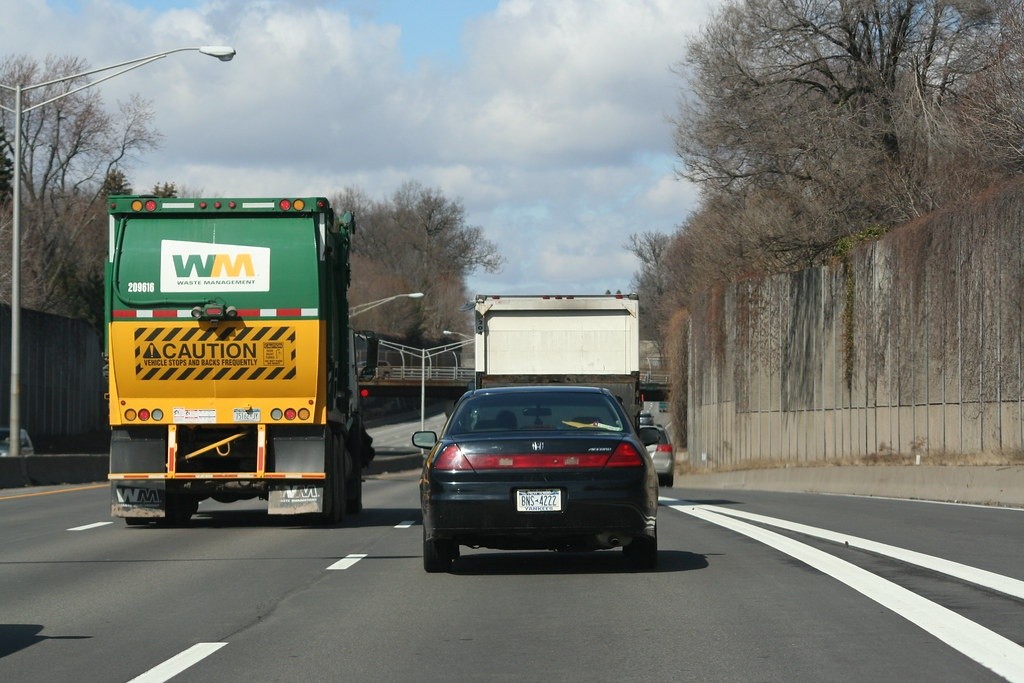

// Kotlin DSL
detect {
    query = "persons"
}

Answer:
[495,410,518,430]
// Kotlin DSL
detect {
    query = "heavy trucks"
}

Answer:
[101,197,381,526]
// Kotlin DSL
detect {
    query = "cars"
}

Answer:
[638,423,674,488]
[410,384,662,573]
[640,412,654,427]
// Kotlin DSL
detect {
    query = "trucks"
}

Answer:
[458,294,643,442]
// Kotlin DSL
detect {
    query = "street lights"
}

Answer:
[1,43,236,457]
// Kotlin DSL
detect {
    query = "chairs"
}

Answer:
[568,415,604,428]
[473,417,511,430]
